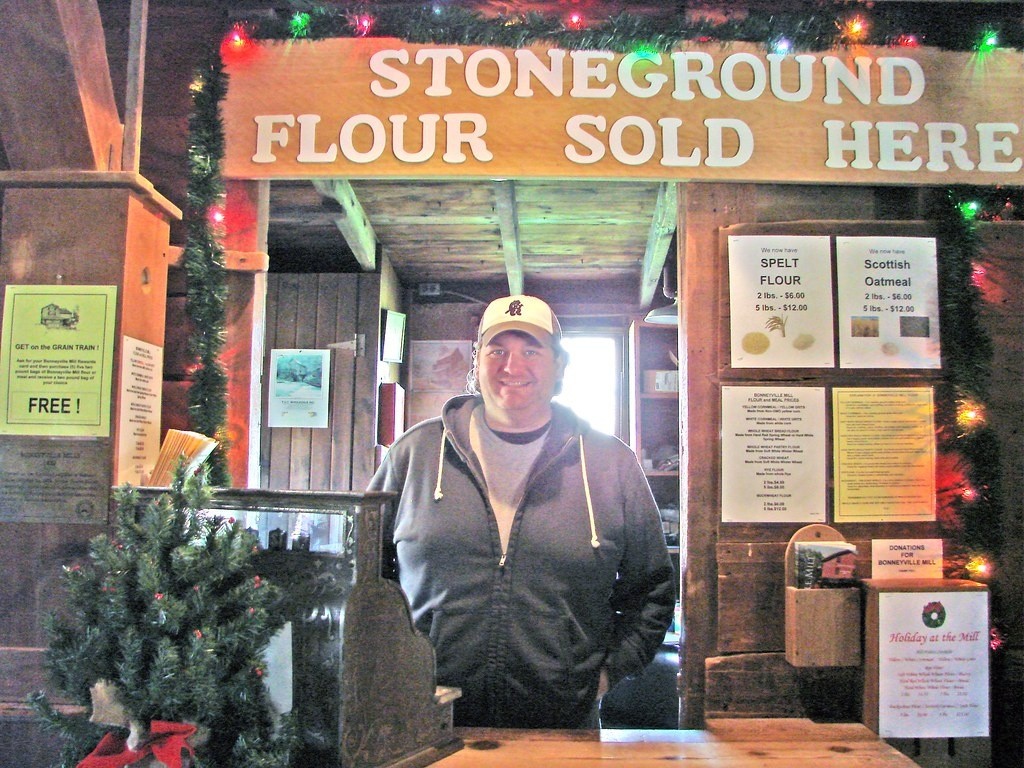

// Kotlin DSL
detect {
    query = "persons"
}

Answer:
[367,294,677,731]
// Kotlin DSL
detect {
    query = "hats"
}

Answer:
[477,294,562,353]
[652,445,679,471]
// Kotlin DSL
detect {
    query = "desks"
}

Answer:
[424,723,922,768]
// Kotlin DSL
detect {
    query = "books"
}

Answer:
[794,542,858,588]
